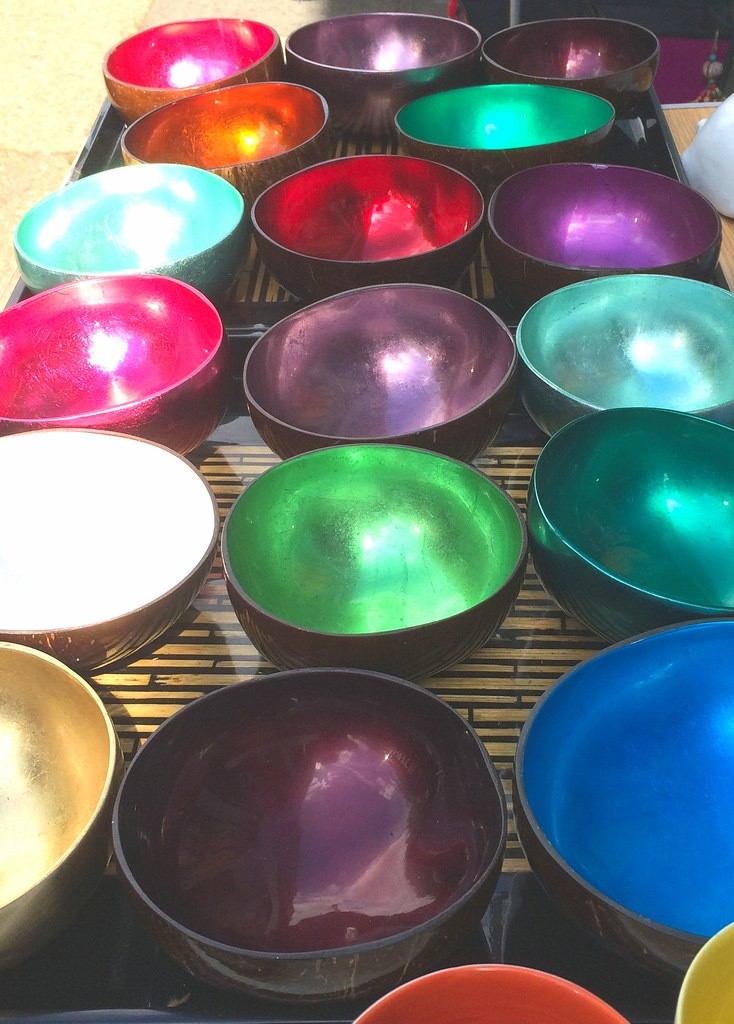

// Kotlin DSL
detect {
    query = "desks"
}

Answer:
[658,93,734,304]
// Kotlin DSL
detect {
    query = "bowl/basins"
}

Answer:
[393,82,617,207]
[249,153,487,305]
[285,11,483,140]
[102,17,285,125]
[514,272,734,437]
[525,406,734,647]
[241,282,520,466]
[487,161,723,318]
[1,273,232,458]
[0,427,222,675]
[11,162,252,316]
[0,615,734,1024]
[120,80,335,232]
[481,16,660,119]
[219,441,530,685]
